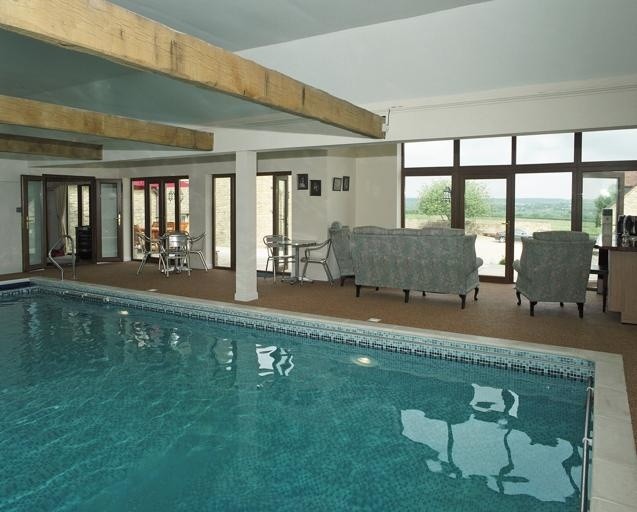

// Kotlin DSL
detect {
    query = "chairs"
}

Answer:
[328,220,380,292]
[261,234,301,284]
[298,238,335,288]
[135,230,210,277]
[511,230,596,321]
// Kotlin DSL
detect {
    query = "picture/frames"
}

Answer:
[296,173,351,196]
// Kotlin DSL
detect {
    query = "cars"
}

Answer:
[495,227,534,243]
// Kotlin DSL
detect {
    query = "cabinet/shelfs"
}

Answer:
[593,246,637,328]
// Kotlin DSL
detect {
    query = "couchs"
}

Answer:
[348,222,484,309]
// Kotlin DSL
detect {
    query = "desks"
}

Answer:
[277,239,317,285]
[574,263,608,313]
